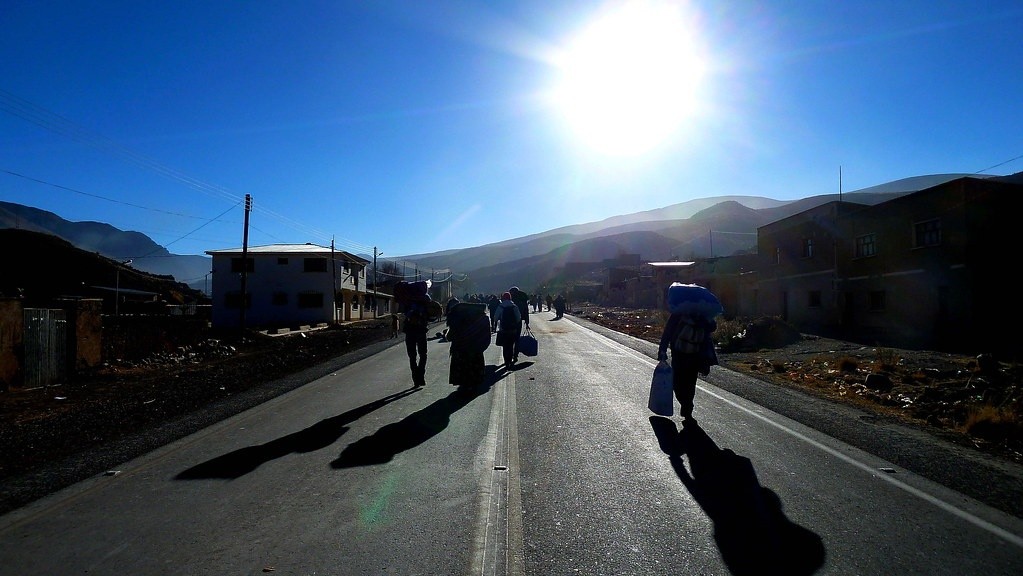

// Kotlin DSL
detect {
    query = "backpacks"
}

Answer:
[499,304,517,331]
[673,315,705,354]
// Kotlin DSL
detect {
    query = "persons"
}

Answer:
[530,293,565,319]
[657,313,716,418]
[446,314,491,385]
[509,286,530,354]
[493,293,521,369]
[435,292,501,333]
[395,280,442,388]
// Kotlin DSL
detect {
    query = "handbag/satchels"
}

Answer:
[518,329,538,356]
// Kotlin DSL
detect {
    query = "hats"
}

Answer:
[502,292,511,300]
